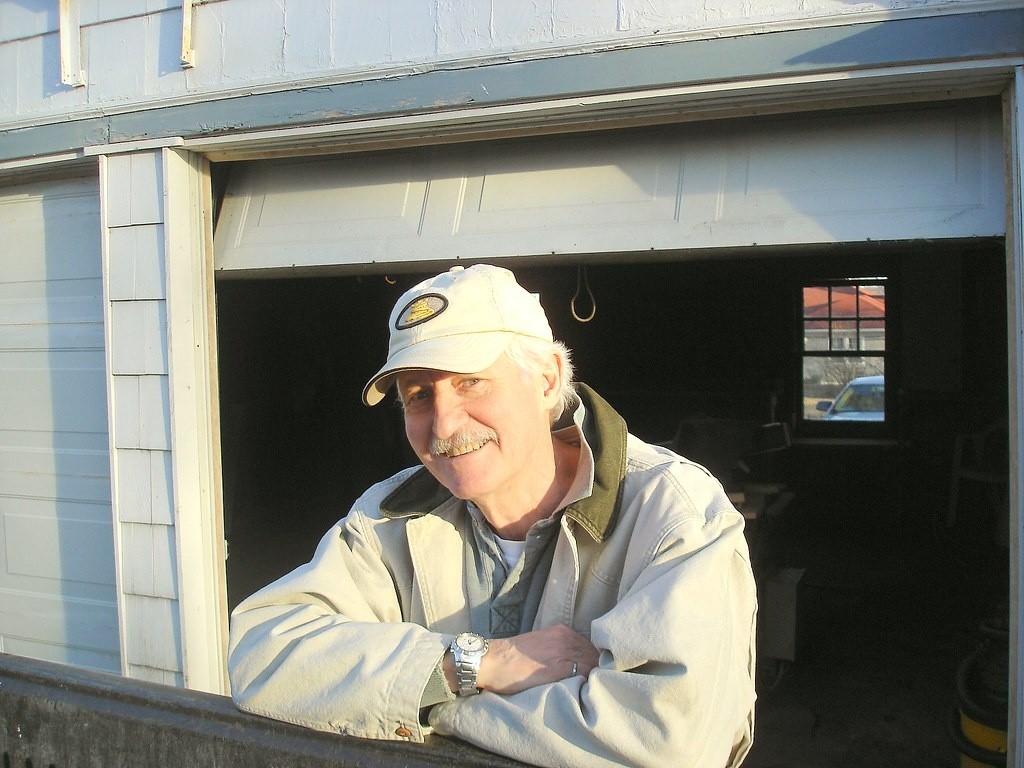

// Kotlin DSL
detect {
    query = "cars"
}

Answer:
[815,374,885,420]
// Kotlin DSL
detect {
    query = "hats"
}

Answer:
[362,263,553,407]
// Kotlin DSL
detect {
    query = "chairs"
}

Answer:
[946,414,1009,549]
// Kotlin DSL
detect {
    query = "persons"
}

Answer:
[229,265,759,768]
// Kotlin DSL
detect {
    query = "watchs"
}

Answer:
[450,632,490,697]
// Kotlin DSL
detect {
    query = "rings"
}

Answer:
[573,662,577,676]
